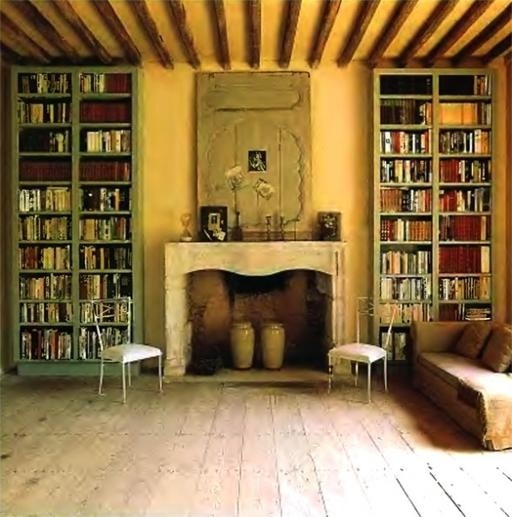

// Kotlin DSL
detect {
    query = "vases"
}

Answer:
[229,320,256,369]
[261,320,286,370]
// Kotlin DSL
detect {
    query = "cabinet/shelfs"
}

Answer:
[372,68,495,376]
[9,65,143,376]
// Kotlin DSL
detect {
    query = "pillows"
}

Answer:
[452,322,493,360]
[480,322,512,372]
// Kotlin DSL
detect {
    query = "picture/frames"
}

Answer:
[318,212,341,240]
[199,206,228,241]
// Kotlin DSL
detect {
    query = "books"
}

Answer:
[380,99,432,360]
[17,74,72,358]
[80,72,132,362]
[439,102,492,321]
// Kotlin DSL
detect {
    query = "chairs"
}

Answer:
[92,296,163,404]
[326,297,400,404]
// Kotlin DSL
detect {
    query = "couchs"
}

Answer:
[410,320,512,451]
